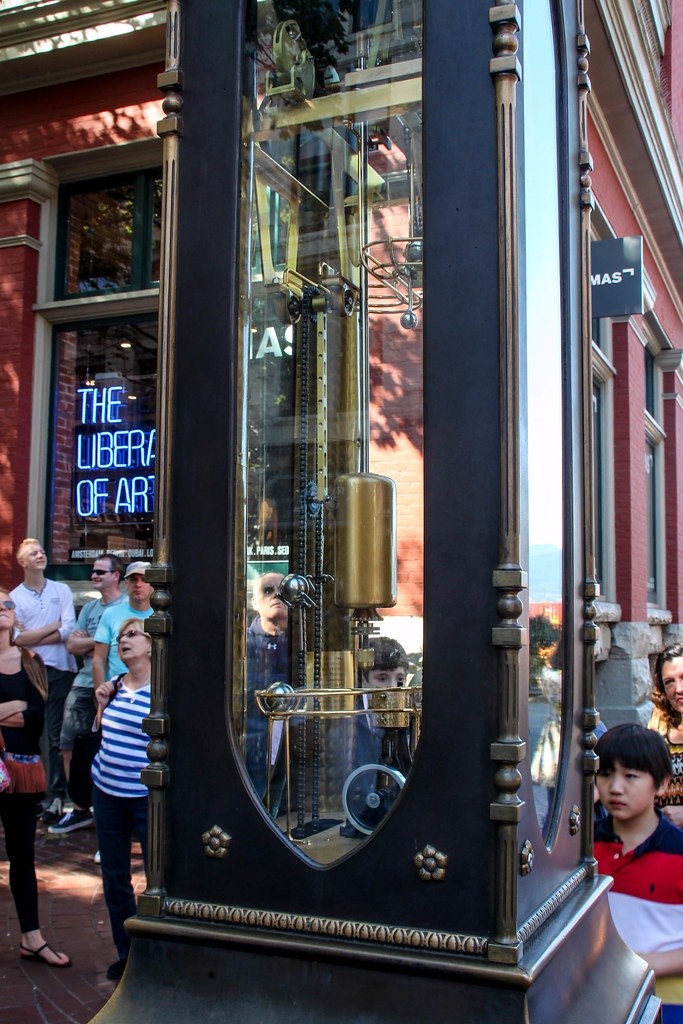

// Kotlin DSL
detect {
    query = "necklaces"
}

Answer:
[125,671,150,703]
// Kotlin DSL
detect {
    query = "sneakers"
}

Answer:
[106,960,127,980]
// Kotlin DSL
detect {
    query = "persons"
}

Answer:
[355,636,410,769]
[7,538,78,823]
[245,573,288,815]
[92,617,152,980]
[648,642,682,831]
[0,591,76,968]
[92,562,155,864]
[594,723,682,1024]
[47,554,130,835]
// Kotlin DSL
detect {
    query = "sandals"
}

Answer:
[19,941,72,968]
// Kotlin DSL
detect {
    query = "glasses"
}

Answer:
[116,629,150,644]
[90,569,116,575]
[0,601,17,610]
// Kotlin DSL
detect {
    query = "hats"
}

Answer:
[123,561,151,579]
